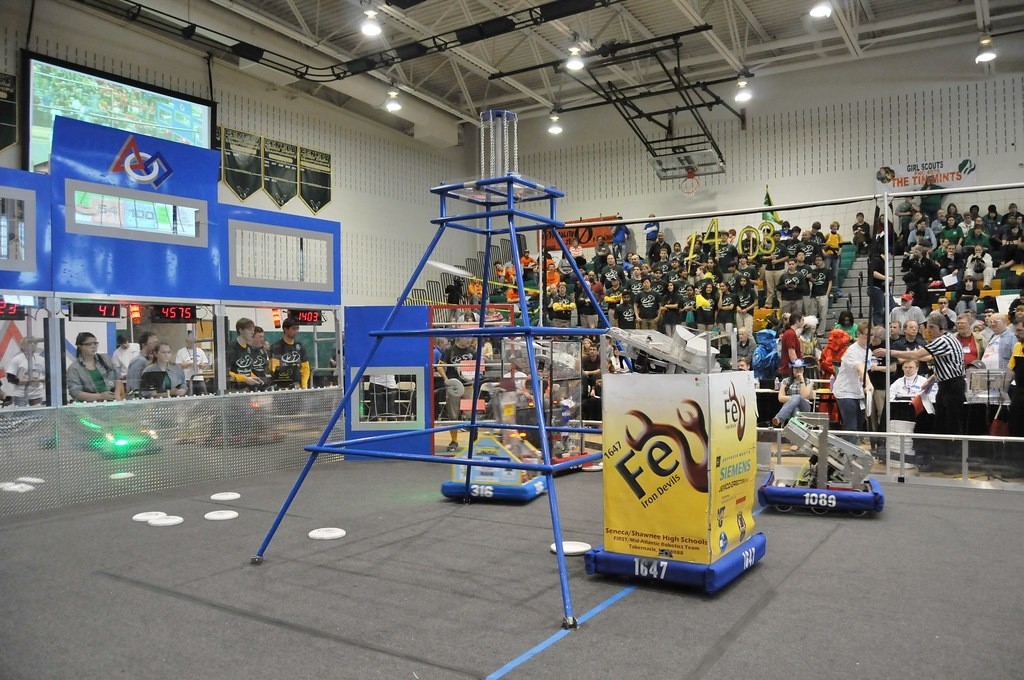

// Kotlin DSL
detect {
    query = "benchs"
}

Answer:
[931,271,1022,311]
[487,264,596,319]
[829,245,858,308]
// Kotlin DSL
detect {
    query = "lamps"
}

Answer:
[387,87,401,110]
[735,76,752,102]
[361,5,381,35]
[567,43,585,70]
[548,113,562,134]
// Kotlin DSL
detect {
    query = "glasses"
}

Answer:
[793,367,804,370]
[79,342,99,347]
[939,302,948,304]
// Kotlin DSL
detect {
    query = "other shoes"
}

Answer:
[942,467,962,474]
[447,442,458,452]
[919,457,945,471]
[835,290,842,297]
[1002,471,1024,477]
[461,427,470,431]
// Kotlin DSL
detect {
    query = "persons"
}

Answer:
[175,330,210,396]
[112,334,141,395]
[145,349,156,366]
[65,332,126,403]
[140,342,189,398]
[263,341,272,361]
[227,317,265,394]
[270,316,310,391]
[126,330,160,401]
[245,326,271,392]
[366,170,1023,485]
[7,335,45,406]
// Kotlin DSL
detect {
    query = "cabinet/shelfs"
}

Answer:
[501,339,583,459]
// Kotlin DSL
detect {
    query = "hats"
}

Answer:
[611,277,620,281]
[558,282,567,286]
[901,294,913,300]
[789,358,806,368]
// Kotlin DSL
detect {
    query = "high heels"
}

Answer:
[772,417,784,428]
